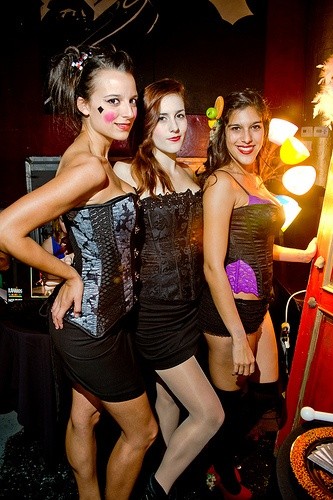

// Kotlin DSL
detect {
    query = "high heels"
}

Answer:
[233,468,242,483]
[207,463,253,500]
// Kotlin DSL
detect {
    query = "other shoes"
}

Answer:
[133,472,169,500]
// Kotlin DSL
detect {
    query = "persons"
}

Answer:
[0,47,158,500]
[112,80,225,500]
[42,214,74,279]
[201,88,317,500]
[0,252,16,291]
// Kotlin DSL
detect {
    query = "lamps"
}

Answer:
[260,118,316,232]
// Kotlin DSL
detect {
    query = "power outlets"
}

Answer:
[301,126,329,138]
